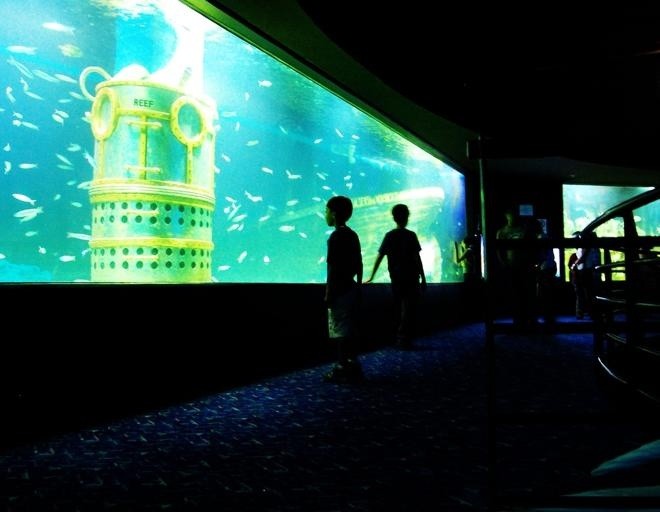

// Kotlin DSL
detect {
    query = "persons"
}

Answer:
[452,210,602,325]
[320,195,369,388]
[365,203,434,353]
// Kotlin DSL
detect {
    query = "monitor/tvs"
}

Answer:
[519,204,534,217]
